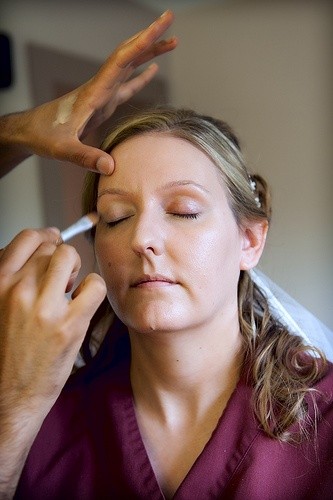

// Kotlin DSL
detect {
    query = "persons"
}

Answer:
[0,9,178,500]
[13,109,333,500]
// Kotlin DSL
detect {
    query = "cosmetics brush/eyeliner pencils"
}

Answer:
[60,212,99,242]
